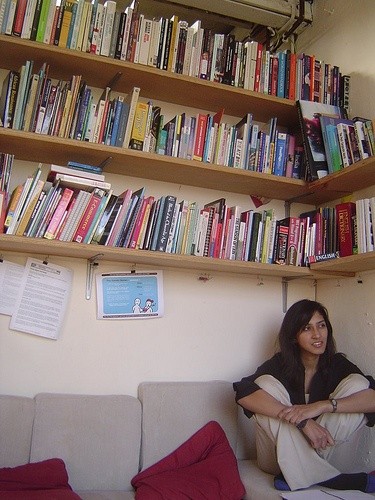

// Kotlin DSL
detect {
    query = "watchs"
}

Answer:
[330,399,338,413]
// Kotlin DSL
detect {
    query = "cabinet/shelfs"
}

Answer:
[0,0,356,281]
[306,154,375,275]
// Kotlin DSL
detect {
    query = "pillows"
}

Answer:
[131,419,247,500]
[0,458,84,500]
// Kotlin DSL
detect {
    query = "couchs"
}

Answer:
[0,378,375,500]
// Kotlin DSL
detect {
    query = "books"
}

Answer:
[1,155,373,265]
[0,0,352,114]
[294,97,375,179]
[0,59,298,177]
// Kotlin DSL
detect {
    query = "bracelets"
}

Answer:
[296,419,308,430]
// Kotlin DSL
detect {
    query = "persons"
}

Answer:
[232,298,375,494]
